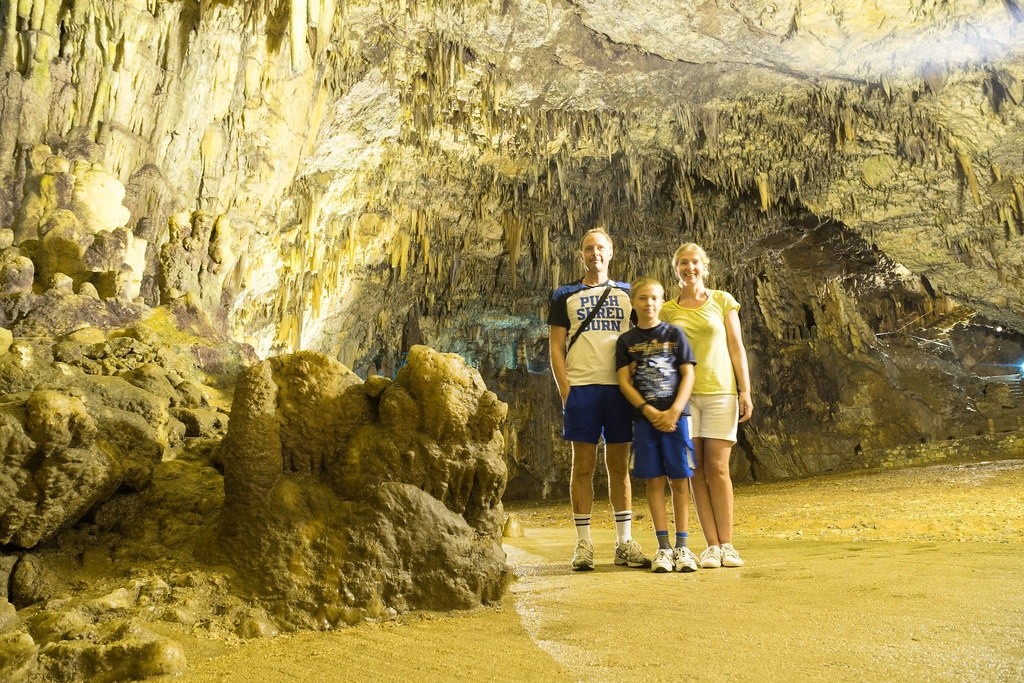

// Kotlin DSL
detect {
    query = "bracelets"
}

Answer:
[637,401,650,413]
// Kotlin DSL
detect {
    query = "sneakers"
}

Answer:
[650,546,677,573]
[698,543,721,567]
[717,542,744,567]
[672,547,700,571]
[568,540,598,571]
[613,542,652,570]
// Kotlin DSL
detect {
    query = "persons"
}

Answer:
[545,226,653,571]
[614,275,701,574]
[659,242,755,567]
